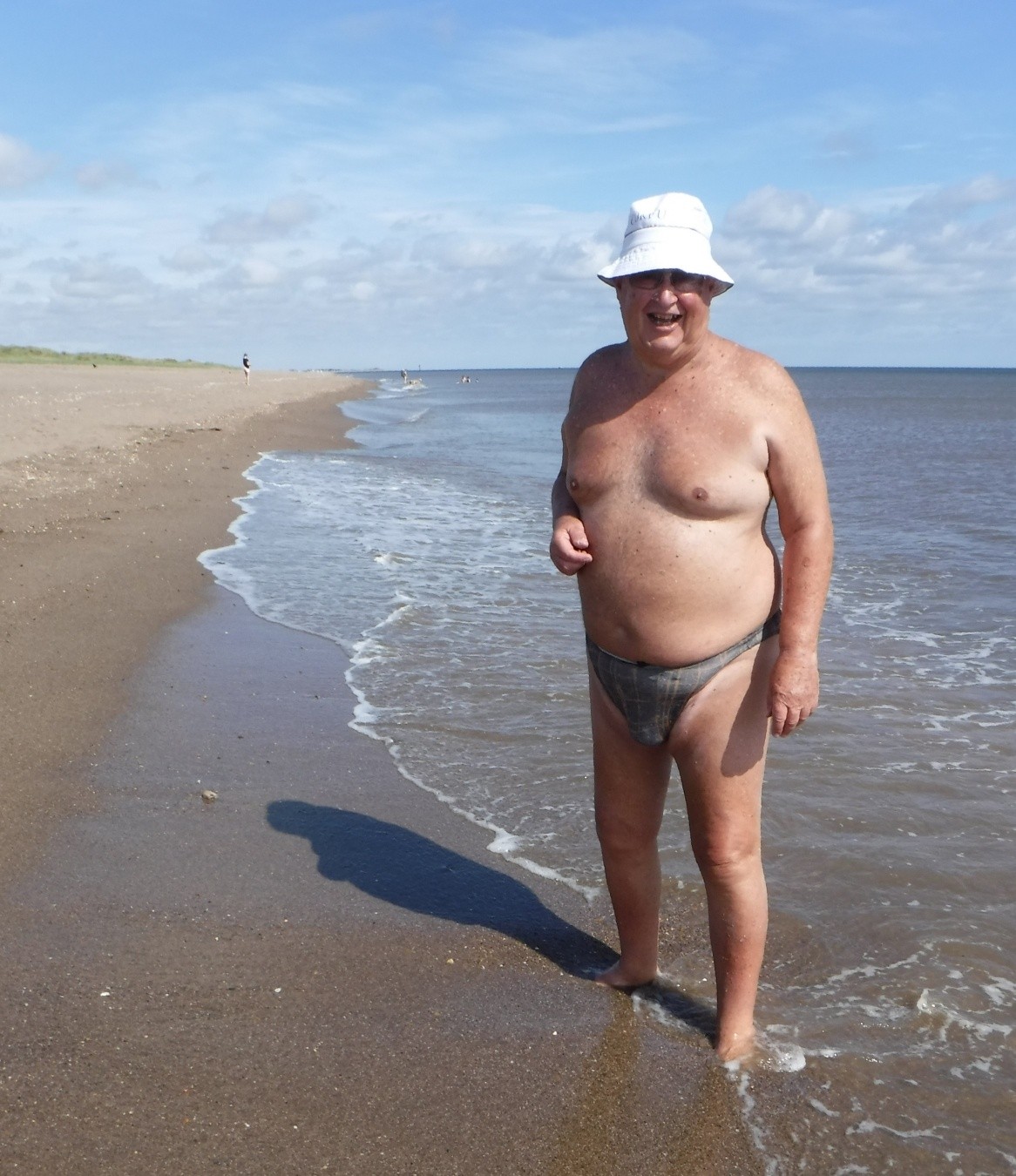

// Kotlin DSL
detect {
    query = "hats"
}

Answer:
[598,193,735,297]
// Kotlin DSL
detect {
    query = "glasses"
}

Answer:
[629,270,707,292]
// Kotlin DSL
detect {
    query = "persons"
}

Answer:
[549,192,835,1062]
[401,369,407,382]
[243,354,251,386]
[462,376,471,383]
